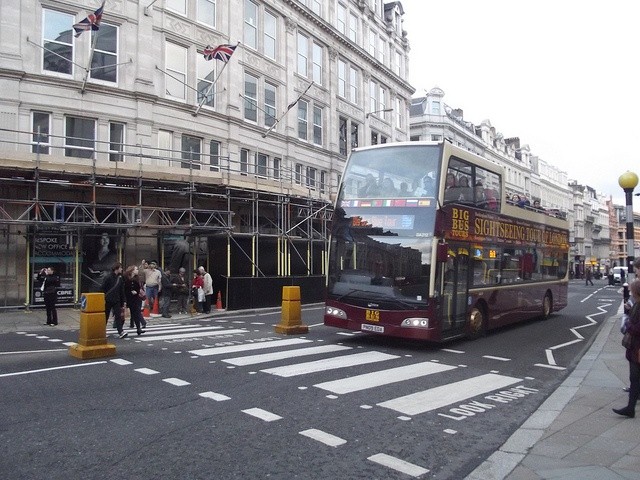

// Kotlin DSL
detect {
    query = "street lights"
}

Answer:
[618,171,639,330]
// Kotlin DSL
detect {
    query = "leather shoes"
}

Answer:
[623,386,629,391]
[162,313,170,318]
[613,407,635,418]
[43,323,47,325]
[51,324,55,326]
[167,313,172,317]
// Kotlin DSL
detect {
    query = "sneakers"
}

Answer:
[120,332,127,338]
[106,335,110,338]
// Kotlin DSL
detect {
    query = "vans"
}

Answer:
[612,266,628,284]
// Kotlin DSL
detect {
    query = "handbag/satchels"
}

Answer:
[139,289,146,301]
[40,278,46,291]
[198,285,207,302]
[622,332,633,348]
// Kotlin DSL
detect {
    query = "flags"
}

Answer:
[200,44,237,64]
[71,6,104,38]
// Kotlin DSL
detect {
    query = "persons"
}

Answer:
[379,177,397,197]
[398,182,411,197]
[144,261,148,268]
[509,194,529,207]
[124,265,146,335]
[612,280,640,417]
[357,174,378,198]
[531,200,545,211]
[624,257,640,313]
[475,182,485,208]
[161,268,176,319]
[101,263,128,339]
[459,175,471,200]
[174,267,189,317]
[37,265,58,327]
[139,258,162,301]
[444,173,459,200]
[421,176,435,196]
[129,303,146,328]
[191,270,204,313]
[584,267,594,285]
[88,232,117,265]
[198,266,213,314]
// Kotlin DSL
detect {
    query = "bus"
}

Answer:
[323,137,576,347]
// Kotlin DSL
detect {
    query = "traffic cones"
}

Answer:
[151,294,162,316]
[213,291,224,311]
[142,296,151,320]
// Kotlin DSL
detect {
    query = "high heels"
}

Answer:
[137,329,146,335]
